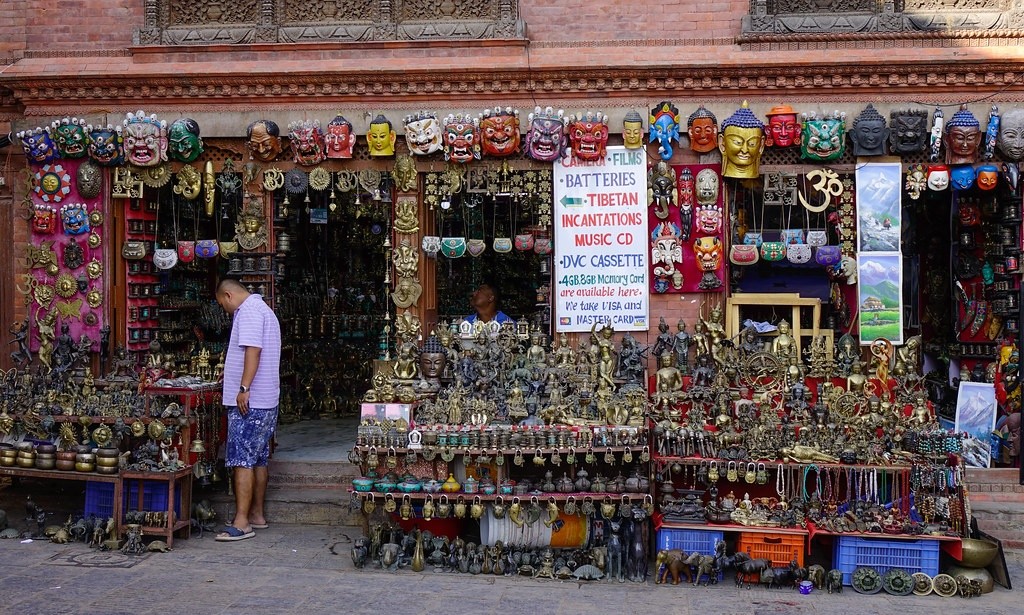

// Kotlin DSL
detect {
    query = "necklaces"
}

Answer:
[775,463,909,515]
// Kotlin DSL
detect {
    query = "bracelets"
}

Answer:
[909,428,972,535]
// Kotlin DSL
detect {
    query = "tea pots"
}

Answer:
[518,467,650,493]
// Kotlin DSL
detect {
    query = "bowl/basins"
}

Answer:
[352,477,374,491]
[462,477,528,495]
[0,441,120,475]
[950,537,998,568]
[397,481,421,492]
[944,566,994,594]
[389,506,461,539]
[374,481,396,493]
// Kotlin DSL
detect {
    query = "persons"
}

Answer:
[743,493,753,509]
[9,304,211,417]
[827,496,838,518]
[394,300,933,447]
[215,278,281,540]
[890,500,900,520]
[462,282,518,327]
[17,101,1024,287]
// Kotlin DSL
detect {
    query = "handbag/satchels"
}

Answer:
[421,236,440,258]
[441,237,466,258]
[534,239,553,254]
[493,238,512,253]
[467,239,486,257]
[153,249,177,269]
[121,242,146,259]
[515,234,535,251]
[729,229,841,266]
[178,241,194,262]
[195,239,219,257]
[219,242,238,260]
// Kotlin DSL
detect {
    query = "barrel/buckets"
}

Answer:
[479,505,591,547]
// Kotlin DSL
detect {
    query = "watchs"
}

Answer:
[239,385,250,393]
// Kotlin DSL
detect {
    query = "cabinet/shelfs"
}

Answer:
[949,184,1020,356]
[124,192,160,352]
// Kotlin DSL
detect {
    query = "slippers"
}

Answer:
[215,526,256,540]
[224,521,268,528]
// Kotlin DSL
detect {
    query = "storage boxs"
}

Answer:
[833,537,939,587]
[655,527,724,582]
[85,479,180,520]
[736,532,806,583]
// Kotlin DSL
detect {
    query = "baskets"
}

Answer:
[656,528,723,582]
[84,478,181,525]
[736,532,805,583]
[832,534,941,587]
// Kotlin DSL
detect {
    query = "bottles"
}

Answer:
[364,467,461,493]
